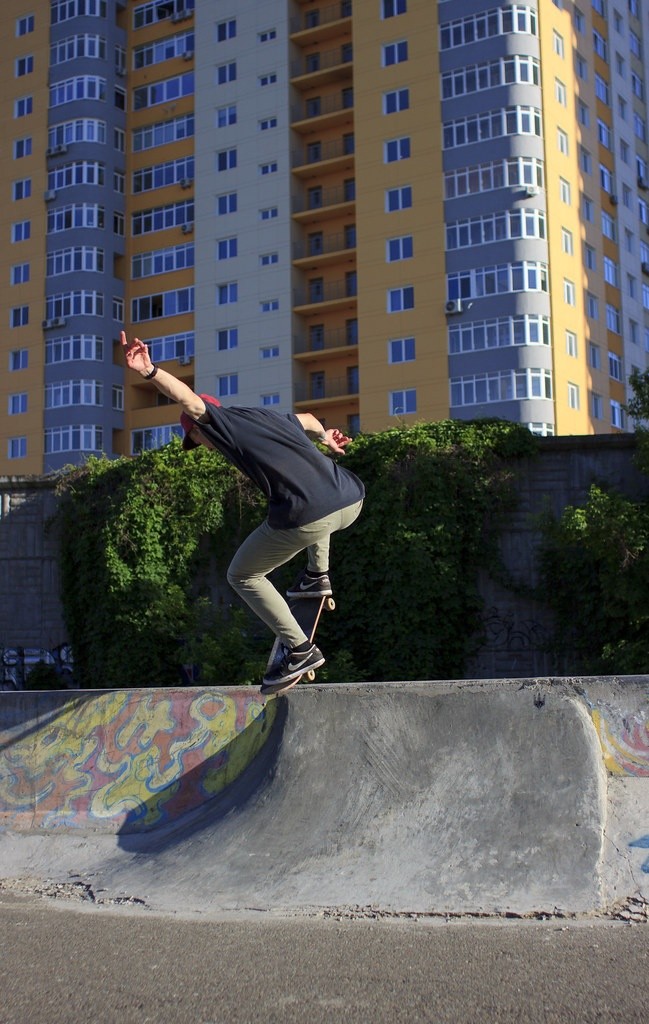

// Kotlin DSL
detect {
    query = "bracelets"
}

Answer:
[144,364,158,380]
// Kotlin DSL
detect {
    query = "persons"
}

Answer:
[121,331,364,686]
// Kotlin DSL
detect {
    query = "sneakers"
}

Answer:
[286,574,333,598]
[262,643,326,685]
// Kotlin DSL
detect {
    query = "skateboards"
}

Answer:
[260,566,336,695]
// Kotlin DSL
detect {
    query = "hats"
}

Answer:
[181,392,222,450]
